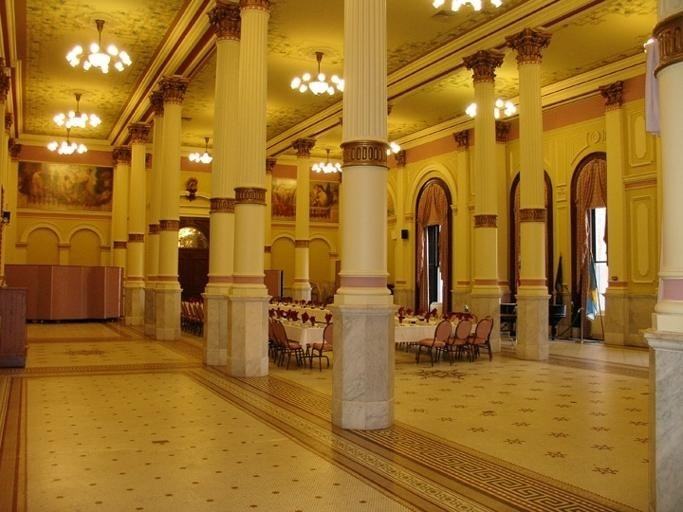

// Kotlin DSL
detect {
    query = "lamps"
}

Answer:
[45,130,89,157]
[188,136,213,165]
[51,90,103,129]
[311,149,343,175]
[64,20,133,76]
[290,50,345,97]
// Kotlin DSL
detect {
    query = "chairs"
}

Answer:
[395,308,492,368]
[270,299,334,370]
[182,300,205,337]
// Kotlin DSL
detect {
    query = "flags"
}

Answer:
[583,255,599,323]
